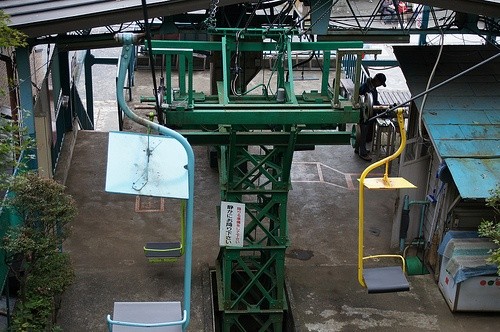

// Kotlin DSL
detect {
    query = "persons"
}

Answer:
[381,0,397,25]
[354,72,387,161]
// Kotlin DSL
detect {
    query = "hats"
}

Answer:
[376,73,386,87]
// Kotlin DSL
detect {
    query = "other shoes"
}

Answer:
[354,148,372,161]
